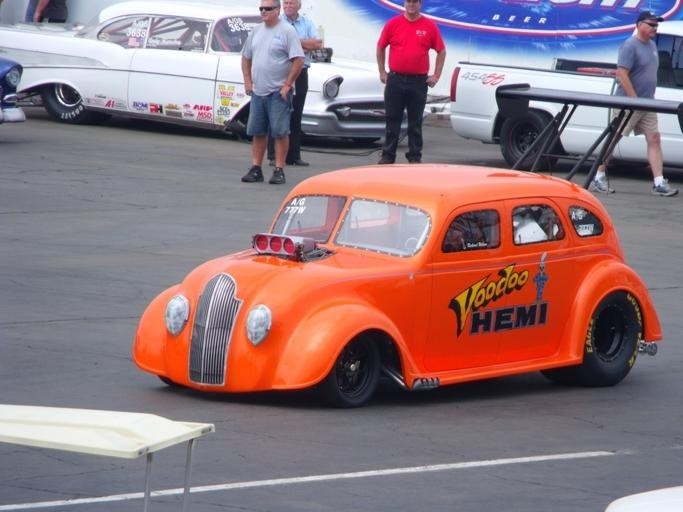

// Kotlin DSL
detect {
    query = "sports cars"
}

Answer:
[0,1,408,148]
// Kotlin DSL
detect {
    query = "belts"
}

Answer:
[389,71,428,78]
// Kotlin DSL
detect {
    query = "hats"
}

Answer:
[636,11,665,23]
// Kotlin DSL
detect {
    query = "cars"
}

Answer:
[132,162,663,409]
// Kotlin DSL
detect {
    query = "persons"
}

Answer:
[375,1,445,164]
[239,1,305,184]
[265,1,322,165]
[590,11,679,197]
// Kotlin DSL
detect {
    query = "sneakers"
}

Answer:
[590,174,615,195]
[650,178,679,196]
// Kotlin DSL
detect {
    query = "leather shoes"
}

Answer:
[241,165,265,183]
[377,156,396,164]
[268,168,286,184]
[286,159,310,165]
[269,159,276,165]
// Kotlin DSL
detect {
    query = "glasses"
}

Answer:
[642,20,659,27]
[259,6,279,11]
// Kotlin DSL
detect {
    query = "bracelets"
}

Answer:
[284,81,290,87]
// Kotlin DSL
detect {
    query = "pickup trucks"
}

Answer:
[450,19,683,172]
